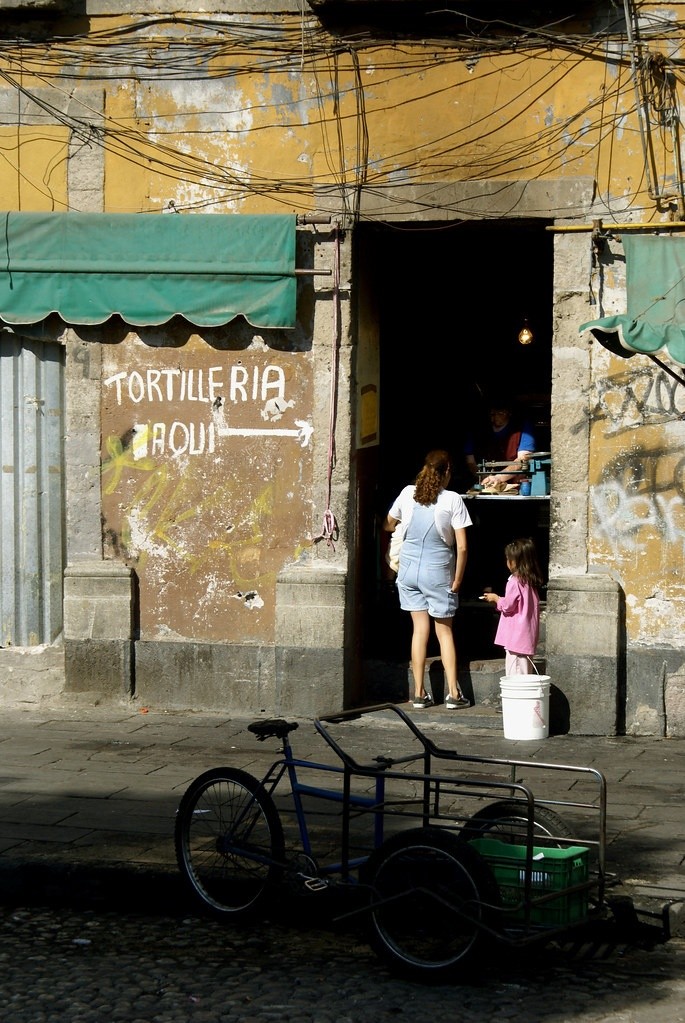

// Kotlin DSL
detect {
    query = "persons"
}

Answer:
[462,397,536,489]
[483,539,540,712]
[382,451,472,709]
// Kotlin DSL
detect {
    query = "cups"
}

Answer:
[484,587,492,597]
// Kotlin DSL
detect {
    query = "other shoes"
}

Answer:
[413,689,434,710]
[495,704,503,713]
[445,695,470,709]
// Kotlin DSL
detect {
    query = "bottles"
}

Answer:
[521,479,530,496]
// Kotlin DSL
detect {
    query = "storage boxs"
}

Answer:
[467,838,591,924]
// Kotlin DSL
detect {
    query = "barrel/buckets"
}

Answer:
[500,653,552,740]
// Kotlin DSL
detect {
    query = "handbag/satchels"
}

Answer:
[384,485,413,573]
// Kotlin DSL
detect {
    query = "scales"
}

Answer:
[474,450,551,496]
